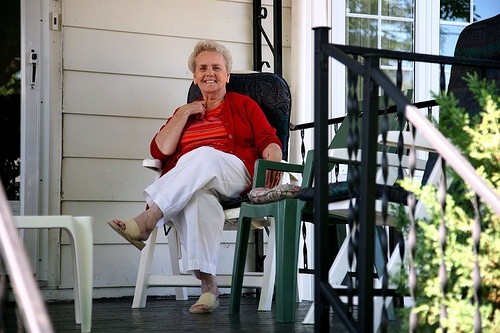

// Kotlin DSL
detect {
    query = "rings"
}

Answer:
[275,176,281,180]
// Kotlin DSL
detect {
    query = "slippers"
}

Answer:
[189,291,220,313]
[107,218,146,251]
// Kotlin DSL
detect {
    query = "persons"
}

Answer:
[106,40,283,313]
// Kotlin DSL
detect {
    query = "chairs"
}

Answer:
[132,72,302,311]
[302,14,500,333]
[229,89,414,322]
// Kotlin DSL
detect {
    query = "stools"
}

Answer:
[11,216,93,333]
